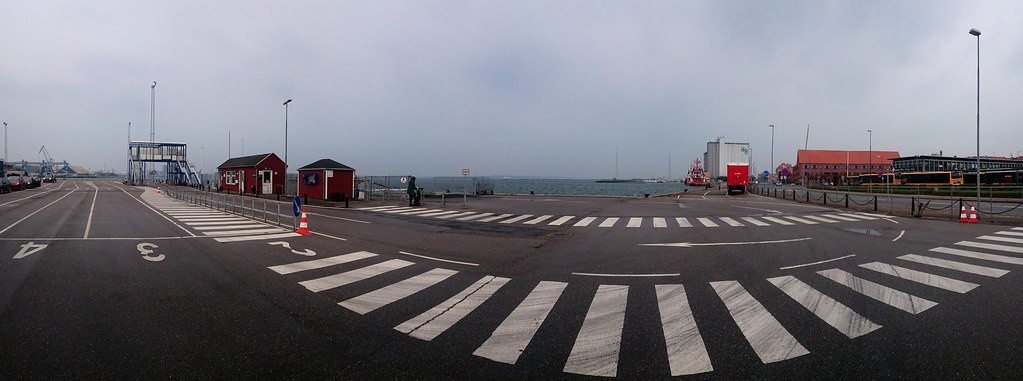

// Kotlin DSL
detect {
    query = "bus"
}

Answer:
[950,171,963,185]
[963,172,985,185]
[896,171,951,186]
[858,174,895,185]
[986,170,1023,185]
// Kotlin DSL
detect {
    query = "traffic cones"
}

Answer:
[959,202,968,223]
[297,208,309,234]
[968,203,978,223]
[157,186,160,193]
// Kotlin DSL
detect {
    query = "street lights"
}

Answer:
[867,129,871,186]
[283,99,292,194]
[969,28,981,218]
[768,125,774,175]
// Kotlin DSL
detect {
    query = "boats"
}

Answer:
[684,158,709,186]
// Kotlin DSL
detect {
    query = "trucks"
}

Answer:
[726,164,749,193]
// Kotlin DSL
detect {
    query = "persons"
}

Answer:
[407,177,417,207]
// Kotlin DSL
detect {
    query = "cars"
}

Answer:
[0,177,12,194]
[43,175,56,183]
[776,181,783,186]
[22,176,35,188]
[7,176,25,191]
[33,177,43,186]
